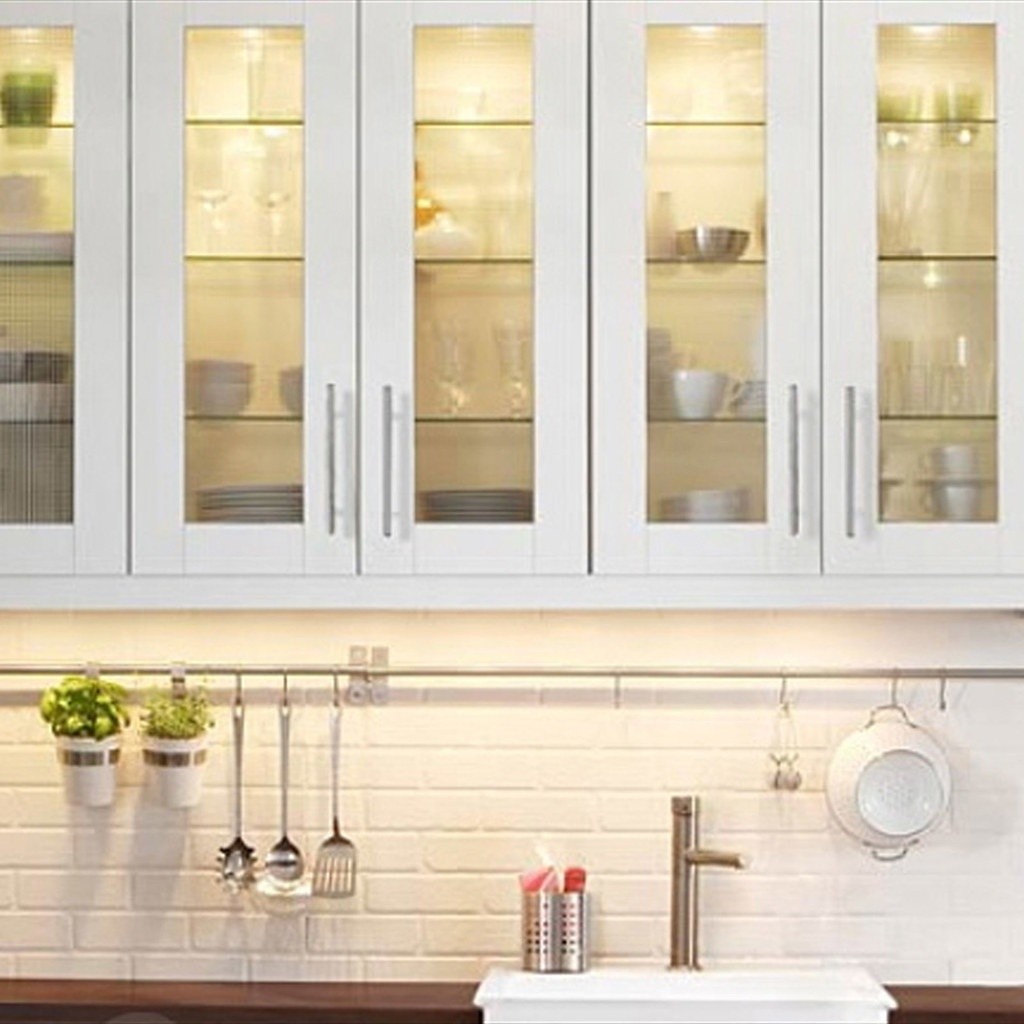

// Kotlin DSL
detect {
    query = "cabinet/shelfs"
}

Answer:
[0,0,1024,615]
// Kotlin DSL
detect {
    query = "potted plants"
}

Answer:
[38,674,133,813]
[134,679,220,813]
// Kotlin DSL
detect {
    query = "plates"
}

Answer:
[659,490,754,520]
[194,482,304,524]
[1,227,76,265]
[417,485,533,523]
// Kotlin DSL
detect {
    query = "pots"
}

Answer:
[825,706,955,861]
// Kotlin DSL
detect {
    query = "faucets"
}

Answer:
[667,795,750,971]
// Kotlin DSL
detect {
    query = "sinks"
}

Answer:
[472,968,899,1023]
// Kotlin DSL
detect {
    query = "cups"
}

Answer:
[914,442,978,478]
[918,484,986,520]
[434,317,465,416]
[879,62,985,142]
[665,371,744,418]
[492,321,527,415]
[0,64,57,128]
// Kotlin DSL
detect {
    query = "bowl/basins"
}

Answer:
[3,349,68,423]
[185,359,304,417]
[676,226,749,259]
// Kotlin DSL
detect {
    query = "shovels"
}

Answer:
[313,706,355,899]
